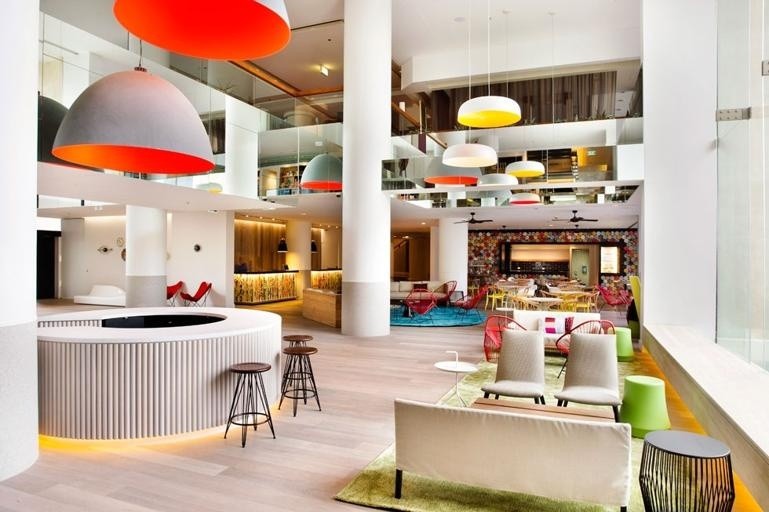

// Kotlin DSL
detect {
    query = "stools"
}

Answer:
[223,362,278,448]
[277,334,317,387]
[277,345,322,418]
[619,374,671,439]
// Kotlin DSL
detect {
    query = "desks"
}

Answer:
[638,429,736,511]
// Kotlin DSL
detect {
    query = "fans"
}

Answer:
[455,212,497,227]
[551,210,597,223]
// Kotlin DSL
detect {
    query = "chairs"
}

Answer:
[435,311,631,421]
[181,282,213,307]
[391,280,635,324]
[166,281,184,306]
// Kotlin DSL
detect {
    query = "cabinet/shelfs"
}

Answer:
[278,165,303,187]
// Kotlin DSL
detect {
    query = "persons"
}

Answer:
[239,256,248,272]
[534,279,549,298]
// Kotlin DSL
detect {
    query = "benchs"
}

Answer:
[392,398,633,512]
[71,284,126,310]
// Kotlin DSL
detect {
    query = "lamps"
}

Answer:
[39,9,105,172]
[112,1,292,65]
[275,221,289,253]
[51,38,216,176]
[420,0,546,204]
[298,152,342,192]
[310,222,318,254]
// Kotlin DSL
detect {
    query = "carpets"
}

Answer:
[330,347,671,512]
